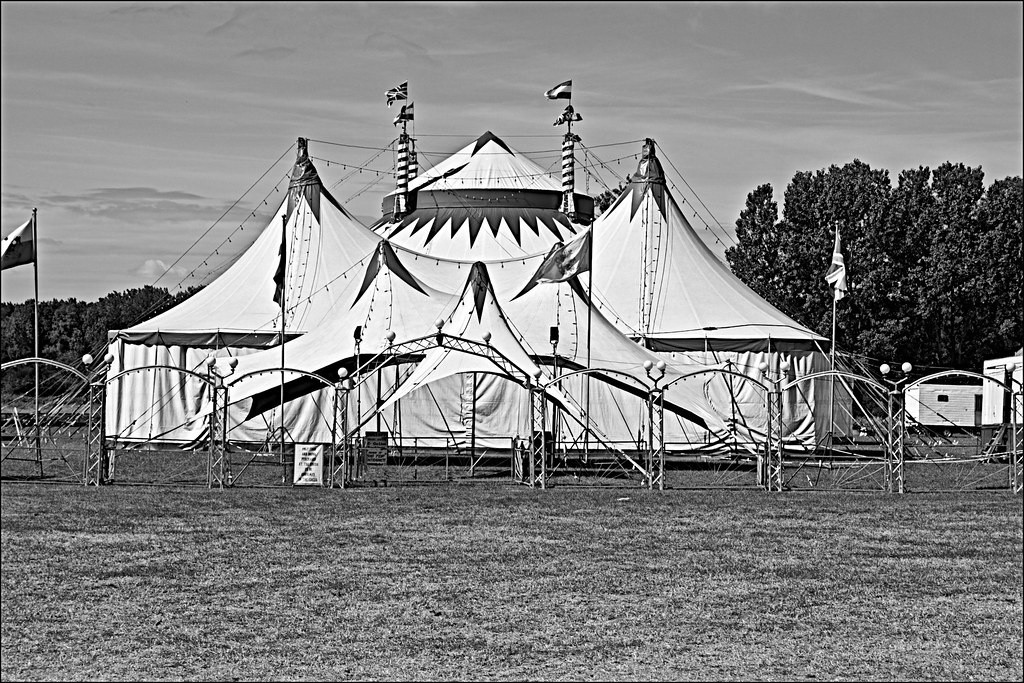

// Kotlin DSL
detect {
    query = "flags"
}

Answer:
[824,225,849,302]
[1,218,36,271]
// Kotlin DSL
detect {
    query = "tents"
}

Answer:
[107,130,854,456]
